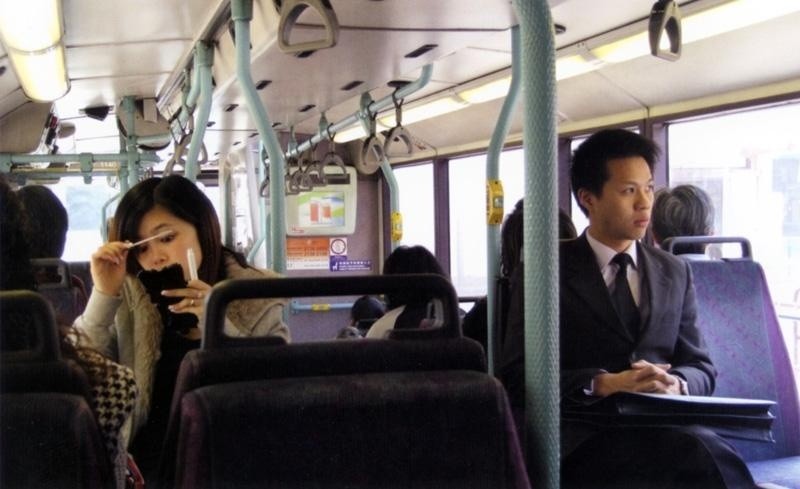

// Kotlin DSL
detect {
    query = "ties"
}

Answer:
[612,253,642,345]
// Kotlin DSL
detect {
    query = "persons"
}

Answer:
[65,174,290,488]
[650,184,715,256]
[461,197,577,341]
[344,245,465,340]
[496,128,760,488]
[1,181,145,487]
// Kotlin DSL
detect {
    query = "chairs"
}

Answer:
[663,235,800,489]
[2,251,531,487]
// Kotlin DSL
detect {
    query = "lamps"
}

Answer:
[0,1,68,101]
[333,0,798,145]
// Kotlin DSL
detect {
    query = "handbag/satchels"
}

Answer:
[558,391,778,444]
